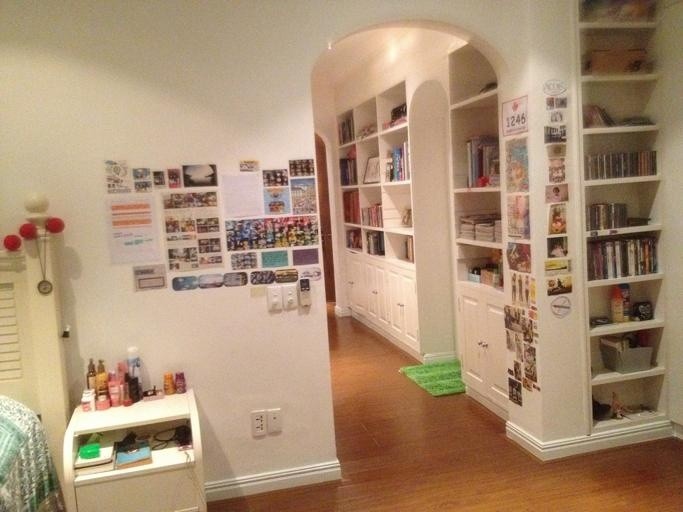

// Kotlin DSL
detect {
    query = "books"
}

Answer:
[382,102,407,128]
[601,335,629,351]
[582,104,653,128]
[468,263,503,288]
[466,134,500,187]
[385,141,410,181]
[405,236,414,262]
[459,214,503,243]
[112,437,152,471]
[588,237,657,281]
[340,117,362,251]
[585,203,651,231]
[585,151,657,180]
[362,203,383,228]
[367,231,385,256]
[73,441,113,469]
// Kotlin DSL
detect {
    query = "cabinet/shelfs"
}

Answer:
[447,44,504,423]
[335,79,421,364]
[577,0,668,434]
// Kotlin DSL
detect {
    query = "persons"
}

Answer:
[545,186,572,285]
[550,168,565,183]
[509,245,531,300]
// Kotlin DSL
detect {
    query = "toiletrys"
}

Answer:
[80,356,141,413]
[86,360,108,397]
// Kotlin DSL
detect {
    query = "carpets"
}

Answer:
[400,358,467,396]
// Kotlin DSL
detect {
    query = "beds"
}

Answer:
[0,188,71,512]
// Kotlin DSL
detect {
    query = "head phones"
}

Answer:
[154,425,188,442]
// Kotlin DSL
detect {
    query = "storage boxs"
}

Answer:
[600,344,653,375]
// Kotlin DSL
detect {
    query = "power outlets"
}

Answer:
[251,410,267,437]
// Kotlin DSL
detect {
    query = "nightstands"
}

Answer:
[63,389,208,512]
